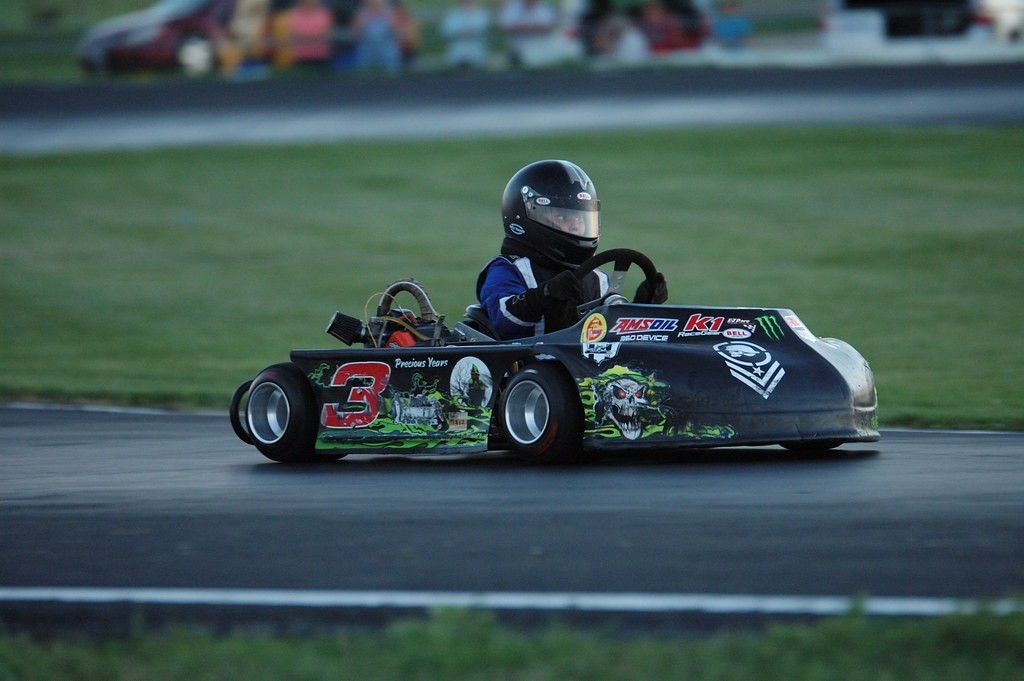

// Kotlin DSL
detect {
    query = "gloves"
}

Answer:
[545,269,580,305]
[636,272,668,304]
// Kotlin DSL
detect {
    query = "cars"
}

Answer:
[77,0,421,75]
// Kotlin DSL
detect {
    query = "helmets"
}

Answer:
[502,159,600,269]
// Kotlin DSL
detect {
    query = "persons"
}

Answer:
[173,0,755,73]
[475,159,669,341]
